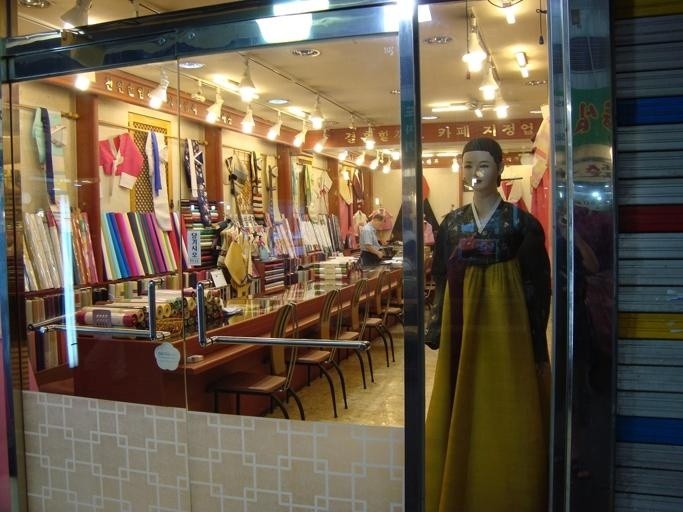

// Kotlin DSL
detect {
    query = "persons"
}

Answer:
[359,214,385,265]
[426,137,552,512]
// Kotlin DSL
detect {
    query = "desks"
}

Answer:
[73,247,436,419]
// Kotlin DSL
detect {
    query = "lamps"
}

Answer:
[369,151,383,170]
[354,150,368,167]
[147,65,170,110]
[60,2,91,32]
[383,155,394,174]
[239,106,257,135]
[313,129,328,155]
[364,127,376,151]
[292,122,307,147]
[492,84,509,120]
[266,114,284,141]
[310,96,326,130]
[452,154,460,173]
[478,63,500,102]
[204,90,225,125]
[236,56,255,103]
[460,18,487,74]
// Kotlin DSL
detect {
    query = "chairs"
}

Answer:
[379,266,436,332]
[318,278,374,390]
[358,270,395,368]
[269,288,348,419]
[203,301,305,421]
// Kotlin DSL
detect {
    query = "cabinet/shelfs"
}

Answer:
[18,79,339,375]
